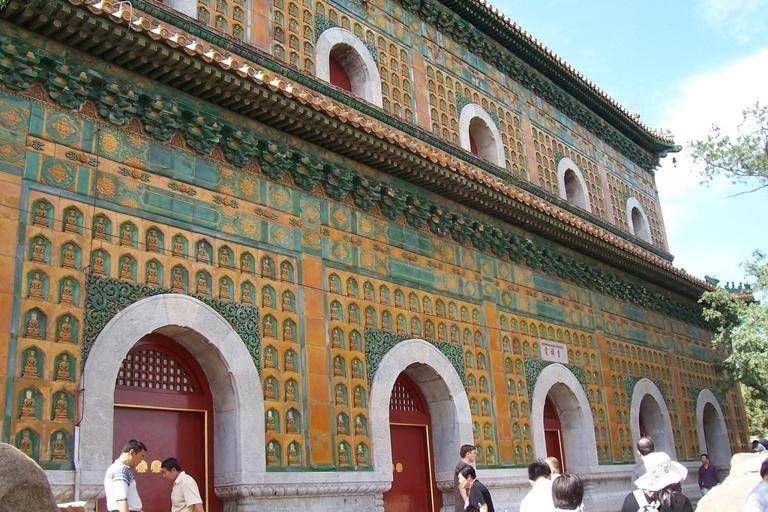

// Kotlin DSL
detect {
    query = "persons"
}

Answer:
[622,451,694,511]
[455,445,477,512]
[1,443,60,512]
[104,439,147,512]
[545,457,561,479]
[742,458,768,512]
[161,458,204,512]
[458,465,495,512]
[630,436,656,491]
[519,460,556,511]
[698,451,721,496]
[552,472,584,511]
[752,439,768,452]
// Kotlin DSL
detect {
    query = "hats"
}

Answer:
[633,451,689,492]
